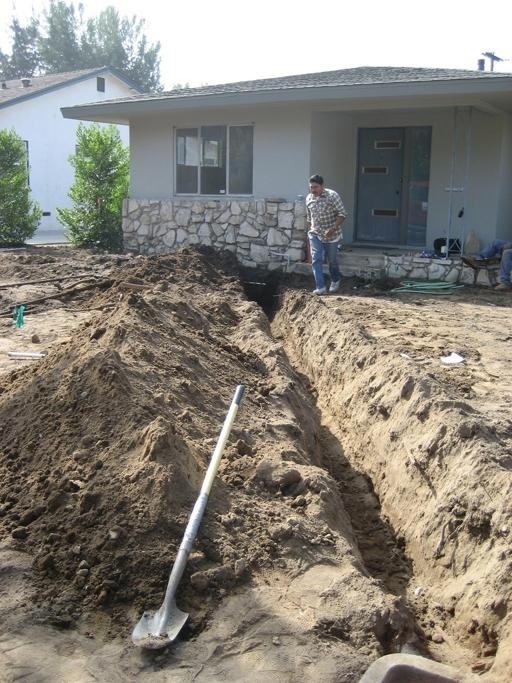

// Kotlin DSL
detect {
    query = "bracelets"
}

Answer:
[332,226,336,231]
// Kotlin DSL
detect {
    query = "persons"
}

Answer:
[305,175,348,295]
[461,238,512,290]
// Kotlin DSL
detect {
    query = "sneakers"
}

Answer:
[329,280,340,292]
[495,283,512,291]
[461,255,478,270]
[313,286,326,294]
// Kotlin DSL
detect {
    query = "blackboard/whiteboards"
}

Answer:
[131,384,245,650]
